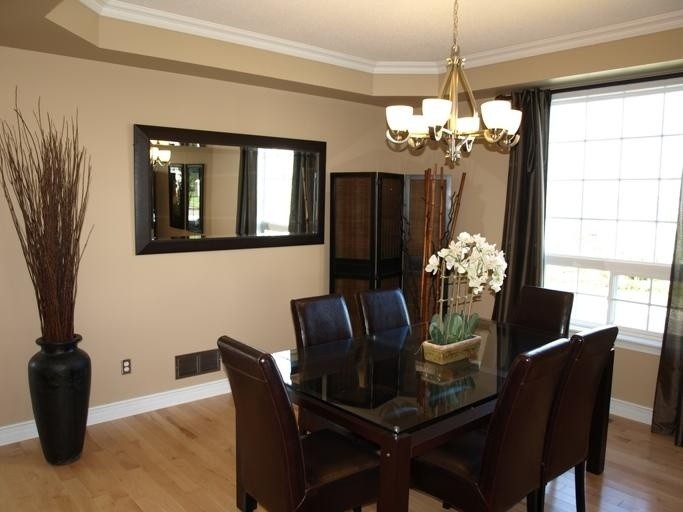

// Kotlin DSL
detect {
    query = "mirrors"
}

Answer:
[135,125,329,254]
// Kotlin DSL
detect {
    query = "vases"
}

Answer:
[422,334,482,365]
[28,335,93,463]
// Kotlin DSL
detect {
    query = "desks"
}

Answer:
[267,310,569,512]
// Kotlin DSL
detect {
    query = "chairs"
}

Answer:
[411,334,583,511]
[508,286,573,336]
[291,295,356,346]
[358,288,412,334]
[218,335,393,512]
[569,327,619,512]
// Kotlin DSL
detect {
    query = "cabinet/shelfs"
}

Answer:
[331,172,451,324]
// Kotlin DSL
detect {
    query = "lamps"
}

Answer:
[146,145,172,168]
[384,0,523,165]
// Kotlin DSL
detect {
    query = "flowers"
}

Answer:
[421,232,508,345]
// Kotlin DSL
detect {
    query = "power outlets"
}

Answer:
[122,358,133,375]
